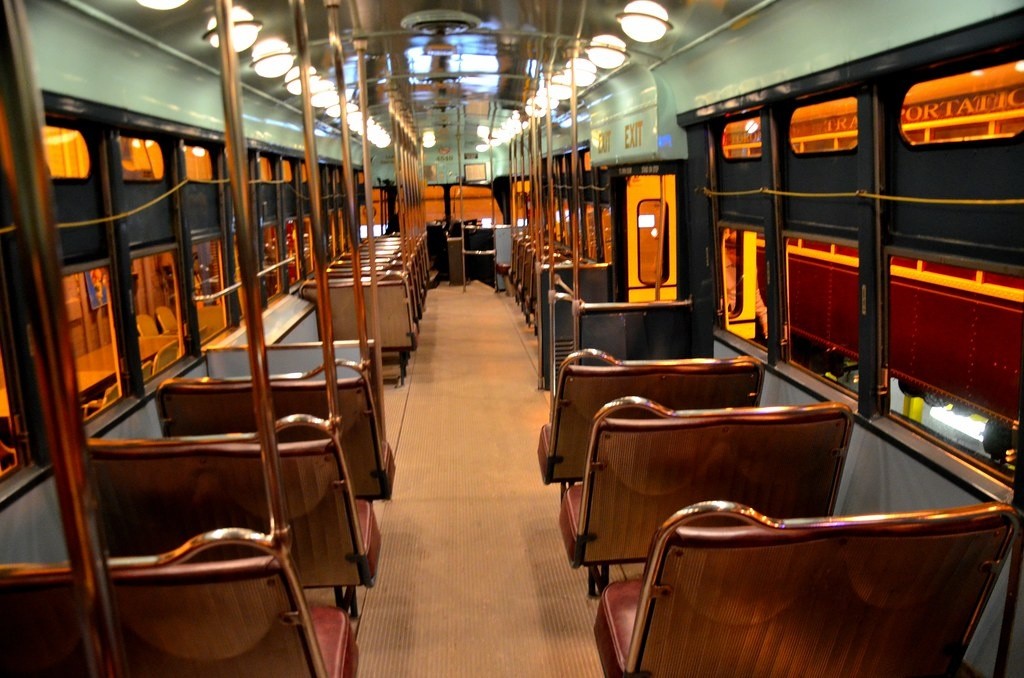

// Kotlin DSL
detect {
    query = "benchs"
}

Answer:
[534,349,1023,677]
[0,233,431,678]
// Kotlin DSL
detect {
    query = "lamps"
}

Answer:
[615,11,673,43]
[281,72,363,124]
[201,21,263,53]
[248,52,298,80]
[536,67,598,111]
[584,45,630,70]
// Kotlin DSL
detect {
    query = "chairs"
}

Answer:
[508,231,596,336]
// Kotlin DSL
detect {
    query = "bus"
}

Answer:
[1,126,503,475]
[718,44,1024,477]
[513,173,758,340]
